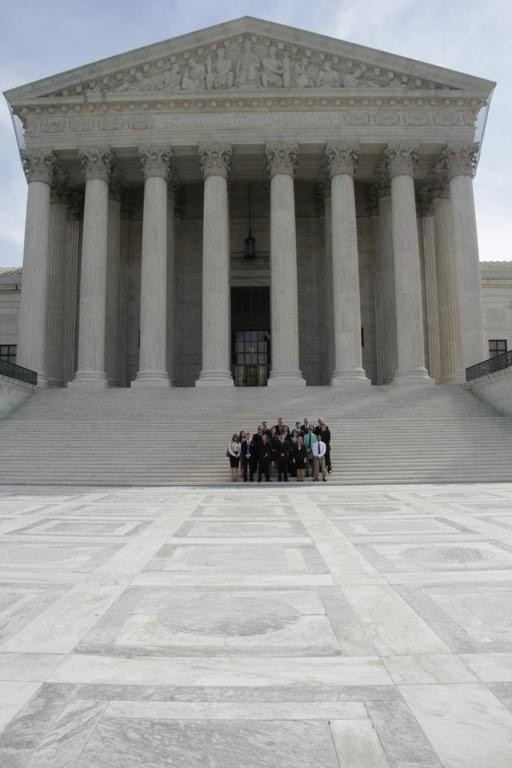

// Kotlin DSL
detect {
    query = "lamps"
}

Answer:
[244,184,256,259]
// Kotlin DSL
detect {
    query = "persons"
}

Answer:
[228,416,332,482]
